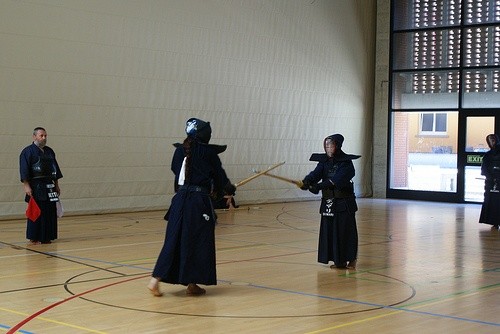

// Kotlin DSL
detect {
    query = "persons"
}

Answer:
[211,193,240,209]
[147,118,237,296]
[479,134,500,231]
[19,127,63,245]
[300,134,361,269]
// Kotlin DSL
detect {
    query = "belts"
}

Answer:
[178,185,208,193]
